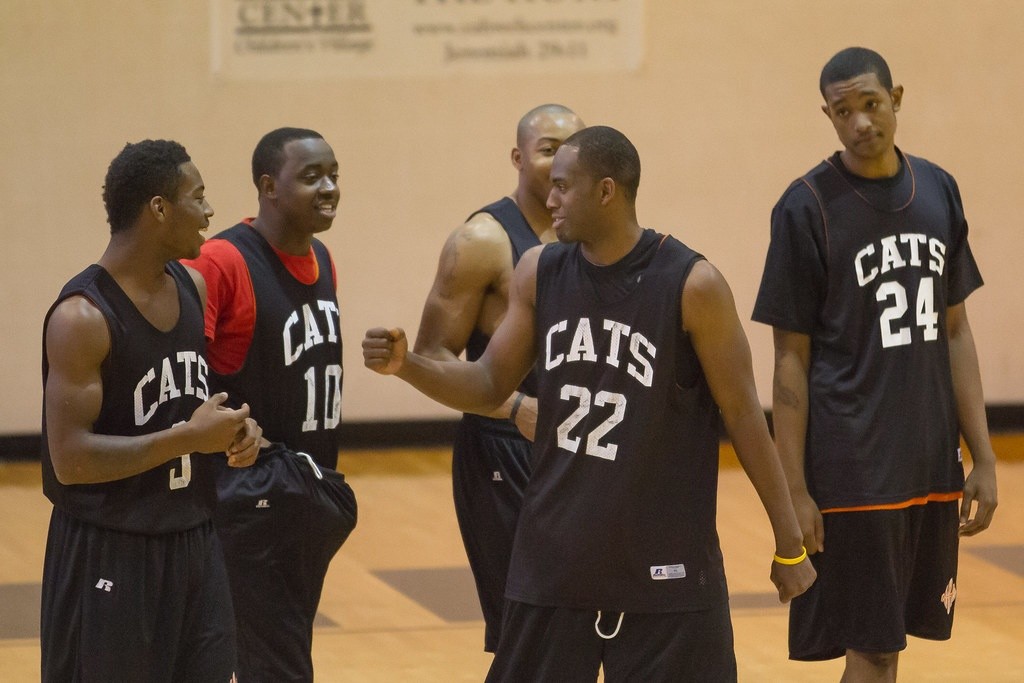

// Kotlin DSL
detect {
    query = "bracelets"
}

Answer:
[509,392,526,425]
[774,546,807,565]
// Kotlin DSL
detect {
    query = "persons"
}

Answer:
[411,105,590,654]
[362,125,817,682]
[179,126,357,683]
[752,47,999,682]
[39,138,264,682]
